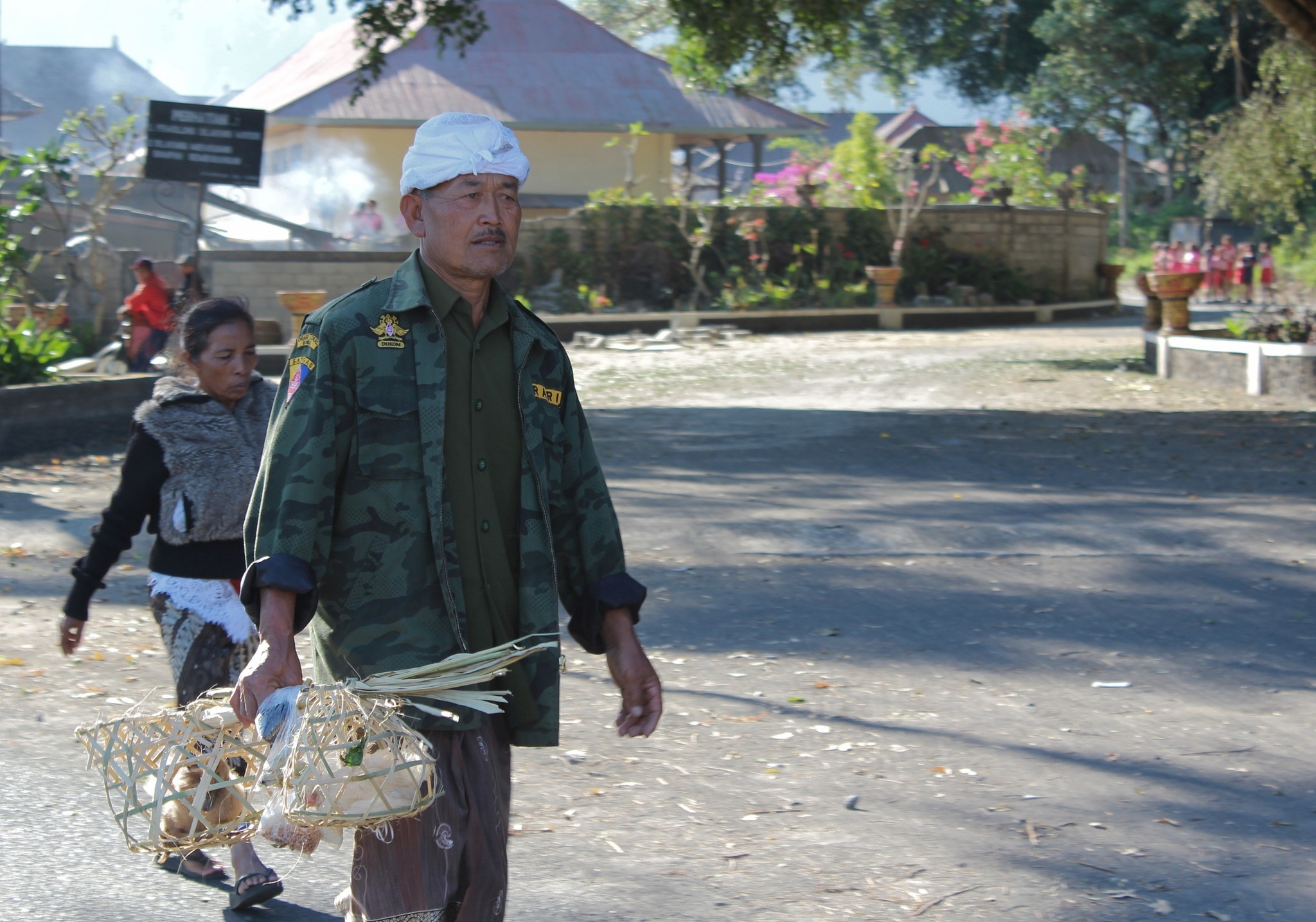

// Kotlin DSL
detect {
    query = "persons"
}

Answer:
[57,295,283,909]
[347,197,386,240]
[118,258,181,374]
[230,113,663,922]
[1145,230,1277,304]
[170,255,213,314]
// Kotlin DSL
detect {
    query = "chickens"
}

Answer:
[302,743,431,814]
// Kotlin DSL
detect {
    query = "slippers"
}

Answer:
[229,868,283,911]
[154,852,224,880]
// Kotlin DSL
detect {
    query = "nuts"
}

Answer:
[262,825,320,854]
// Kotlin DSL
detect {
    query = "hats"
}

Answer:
[400,112,530,196]
[128,258,154,271]
[174,254,197,265]
